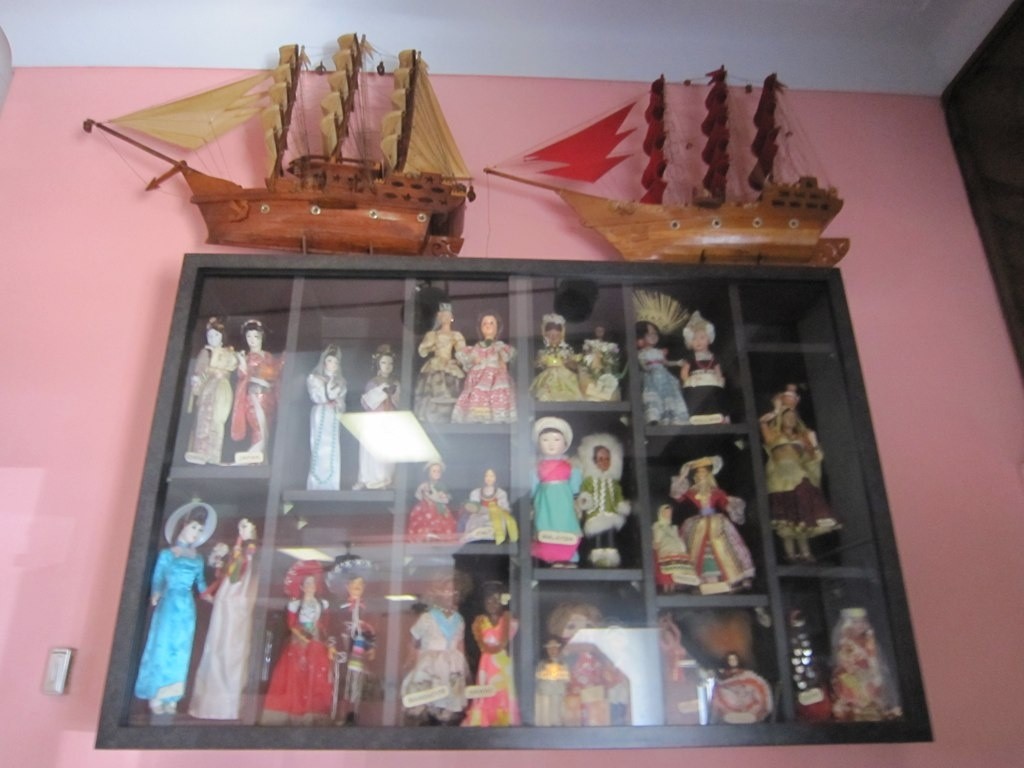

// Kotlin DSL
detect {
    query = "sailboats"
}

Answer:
[482,63,853,268]
[78,32,482,262]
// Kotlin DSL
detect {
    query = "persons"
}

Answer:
[531,311,584,403]
[529,415,584,570]
[306,341,347,491]
[131,300,903,731]
[758,380,845,563]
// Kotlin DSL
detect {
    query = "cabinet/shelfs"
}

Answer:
[95,253,936,750]
